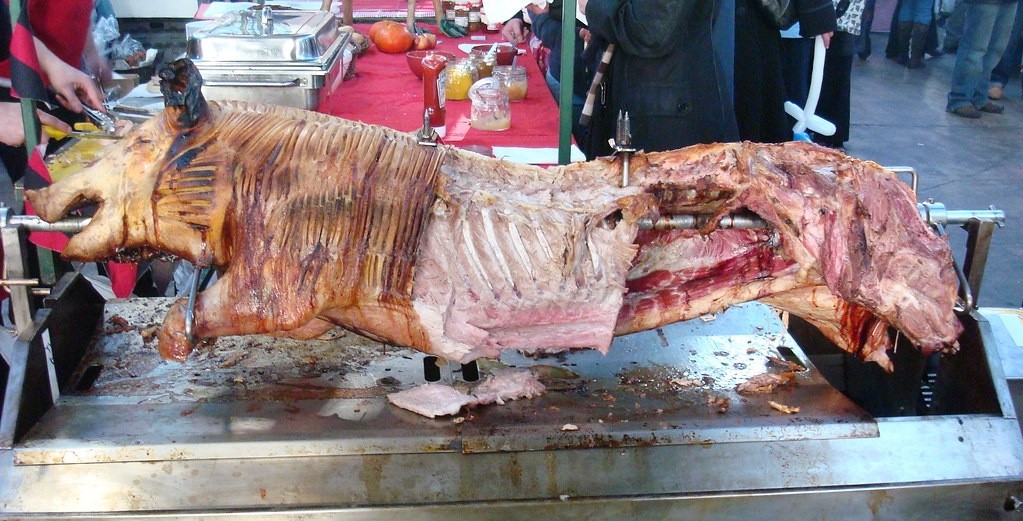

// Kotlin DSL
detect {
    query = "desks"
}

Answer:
[318,0,587,170]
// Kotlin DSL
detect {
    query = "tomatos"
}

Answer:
[369,20,413,54]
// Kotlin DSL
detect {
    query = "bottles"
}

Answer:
[419,54,448,140]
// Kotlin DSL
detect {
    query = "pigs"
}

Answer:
[24,57,966,375]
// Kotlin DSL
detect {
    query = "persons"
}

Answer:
[501,0,1023,162]
[0,0,120,150]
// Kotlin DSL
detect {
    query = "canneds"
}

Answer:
[441,0,500,34]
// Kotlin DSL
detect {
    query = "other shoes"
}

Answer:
[922,45,946,57]
[987,85,1004,100]
[979,102,1005,114]
[952,105,981,118]
[905,59,927,69]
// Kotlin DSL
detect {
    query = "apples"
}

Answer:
[413,33,437,50]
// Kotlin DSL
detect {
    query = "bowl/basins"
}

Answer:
[407,51,456,81]
[471,45,518,65]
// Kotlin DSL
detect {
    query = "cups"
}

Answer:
[445,58,528,131]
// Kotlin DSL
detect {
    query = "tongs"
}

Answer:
[45,85,116,132]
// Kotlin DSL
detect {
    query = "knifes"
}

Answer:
[71,132,125,139]
